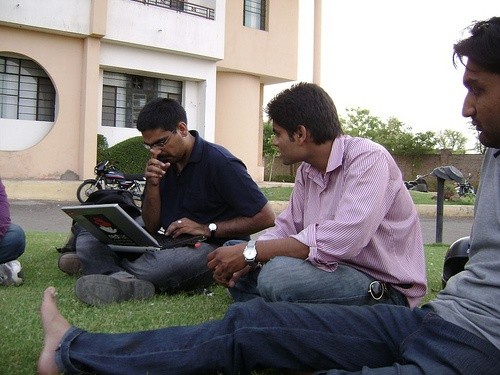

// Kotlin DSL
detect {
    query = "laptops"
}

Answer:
[61,203,207,252]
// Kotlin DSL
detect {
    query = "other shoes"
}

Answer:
[73,270,157,306]
[57,252,79,274]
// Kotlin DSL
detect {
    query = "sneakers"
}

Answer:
[0,260,25,287]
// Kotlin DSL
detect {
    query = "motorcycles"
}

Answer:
[404,174,430,193]
[76,160,146,209]
[454,172,476,195]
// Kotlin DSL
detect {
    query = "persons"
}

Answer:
[0,177,25,288]
[36,17,500,375]
[206,82,427,308]
[59,98,276,306]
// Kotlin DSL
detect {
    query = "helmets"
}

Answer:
[441,235,474,290]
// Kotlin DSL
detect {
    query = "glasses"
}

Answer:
[143,125,179,150]
[368,280,392,299]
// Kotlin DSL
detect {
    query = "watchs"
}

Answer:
[242,239,257,269]
[208,219,218,240]
